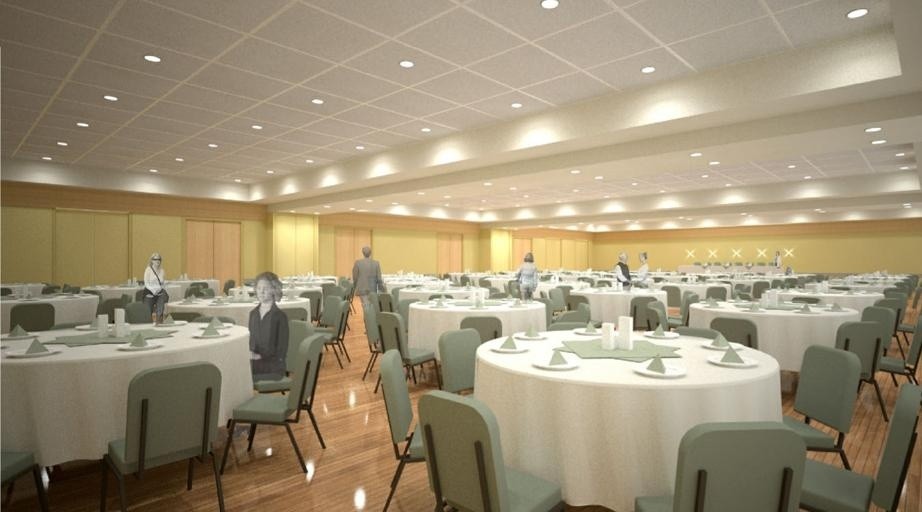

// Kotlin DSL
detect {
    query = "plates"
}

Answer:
[514,333,545,339]
[634,361,686,375]
[645,331,679,337]
[76,325,126,329]
[193,332,229,337]
[119,343,161,348]
[153,321,188,325]
[702,340,744,348]
[706,355,755,365]
[201,323,233,327]
[492,346,528,352]
[534,360,579,368]
[6,348,58,356]
[575,327,608,334]
[1,332,39,338]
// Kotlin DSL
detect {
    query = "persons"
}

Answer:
[615,252,631,286]
[774,251,782,268]
[786,268,792,275]
[249,272,288,381]
[516,253,538,298]
[352,247,385,335]
[142,251,169,323]
[632,251,649,282]
